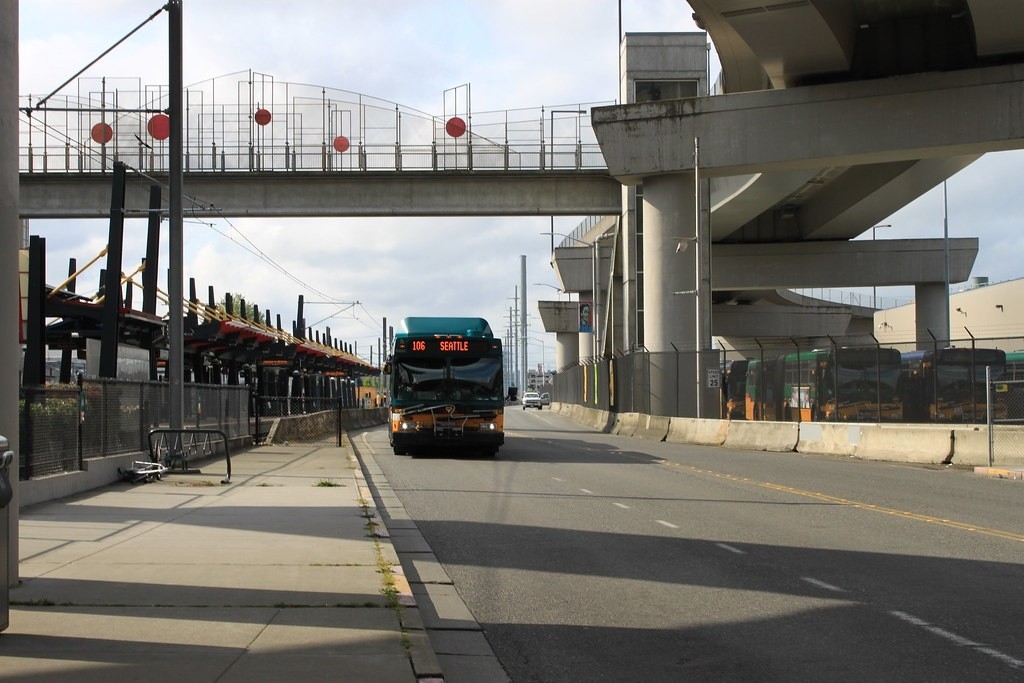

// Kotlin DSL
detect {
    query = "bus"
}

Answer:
[745,347,904,423]
[722,360,749,420]
[901,348,1008,423]
[383,316,518,460]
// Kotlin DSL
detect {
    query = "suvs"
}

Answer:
[522,392,550,410]
